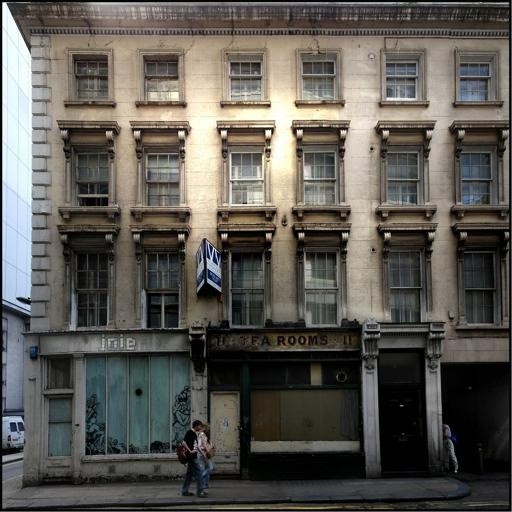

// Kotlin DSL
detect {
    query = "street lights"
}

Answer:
[16,297,31,305]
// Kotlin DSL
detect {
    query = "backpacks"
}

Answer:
[449,424,458,445]
[175,440,189,464]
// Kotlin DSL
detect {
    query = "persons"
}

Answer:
[181,420,208,498]
[443,424,459,474]
[196,423,213,495]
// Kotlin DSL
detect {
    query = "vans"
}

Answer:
[2,415,24,452]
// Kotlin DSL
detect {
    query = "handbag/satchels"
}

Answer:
[206,448,216,459]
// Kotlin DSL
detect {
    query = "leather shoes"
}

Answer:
[180,491,208,497]
